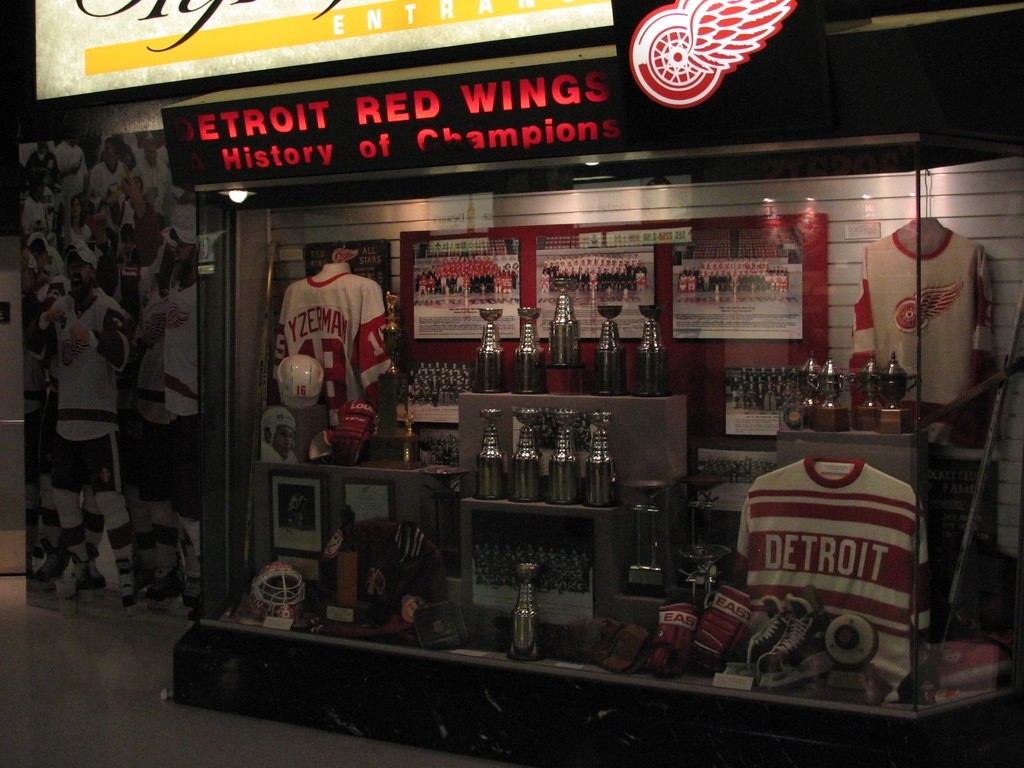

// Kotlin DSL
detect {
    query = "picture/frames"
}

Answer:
[271,552,322,587]
[340,478,396,524]
[269,469,329,558]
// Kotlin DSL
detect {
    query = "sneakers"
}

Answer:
[87,558,107,594]
[146,567,178,602]
[759,588,840,688]
[119,569,137,617]
[181,576,203,608]
[745,590,792,670]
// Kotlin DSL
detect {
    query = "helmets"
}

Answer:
[261,408,296,443]
[276,355,324,408]
[250,560,306,623]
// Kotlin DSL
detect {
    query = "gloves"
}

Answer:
[648,602,699,680]
[695,587,752,675]
[331,400,376,462]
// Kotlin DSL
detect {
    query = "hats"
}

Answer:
[28,232,49,253]
[169,224,199,251]
[161,227,179,248]
[64,239,88,252]
[66,248,97,270]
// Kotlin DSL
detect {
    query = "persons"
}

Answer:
[738,457,931,669]
[22,138,203,607]
[261,405,299,463]
[733,367,815,413]
[409,362,471,407]
[287,492,313,530]
[855,218,993,404]
[413,253,788,304]
[273,264,391,430]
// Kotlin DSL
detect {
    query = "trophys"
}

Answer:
[380,292,401,375]
[476,296,670,660]
[800,348,922,412]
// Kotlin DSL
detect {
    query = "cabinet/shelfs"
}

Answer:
[261,391,923,634]
